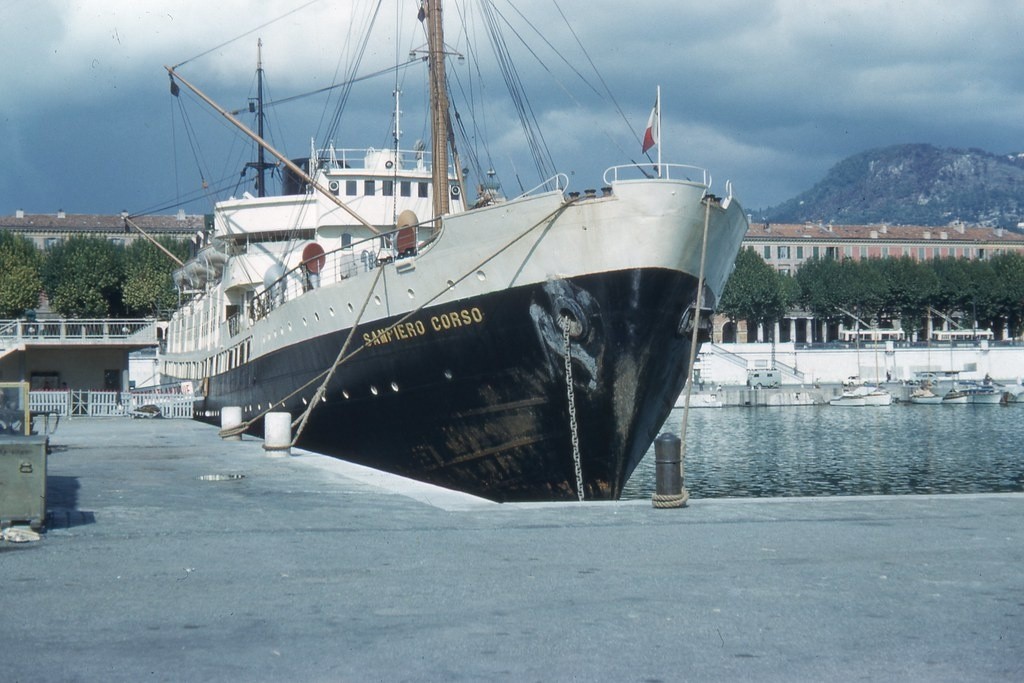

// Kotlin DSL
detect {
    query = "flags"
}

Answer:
[642,86,661,154]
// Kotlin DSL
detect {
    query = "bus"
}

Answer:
[932,329,994,340]
[842,329,905,341]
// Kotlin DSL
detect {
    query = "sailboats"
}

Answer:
[829,325,892,406]
[908,309,943,404]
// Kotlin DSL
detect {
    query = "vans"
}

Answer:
[747,370,781,389]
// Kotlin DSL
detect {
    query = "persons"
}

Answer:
[698,376,722,392]
[61,383,68,392]
[814,378,820,389]
[42,380,51,391]
[886,370,892,383]
[984,372,990,385]
[25,304,36,335]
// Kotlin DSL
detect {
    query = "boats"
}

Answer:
[943,381,1024,404]
[156,0,750,501]
